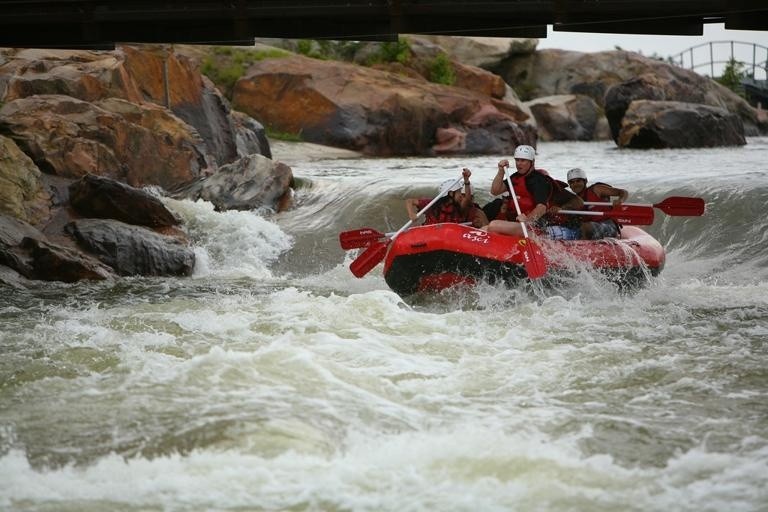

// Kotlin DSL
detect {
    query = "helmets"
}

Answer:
[567,168,586,183]
[440,179,463,198]
[514,145,535,161]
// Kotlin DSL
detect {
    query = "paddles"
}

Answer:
[558,205,654,226]
[339,169,468,278]
[502,165,547,280]
[584,196,705,217]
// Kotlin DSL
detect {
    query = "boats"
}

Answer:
[382,202,666,310]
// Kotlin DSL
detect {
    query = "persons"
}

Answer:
[406,144,629,241]
